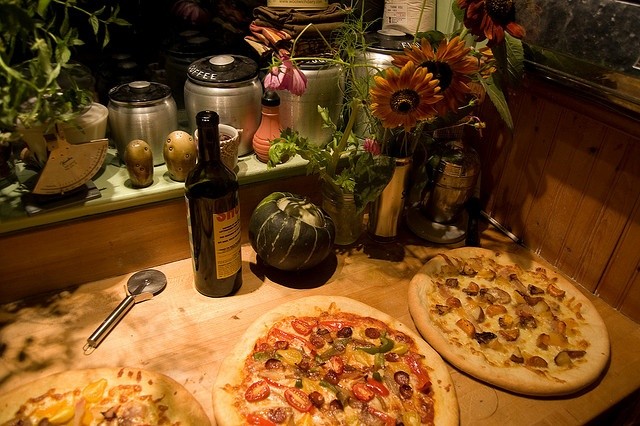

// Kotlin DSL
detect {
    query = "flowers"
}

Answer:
[363,34,479,158]
[446,0,534,160]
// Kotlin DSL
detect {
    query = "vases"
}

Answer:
[260,17,395,215]
[421,156,479,224]
[369,158,412,243]
[321,184,365,246]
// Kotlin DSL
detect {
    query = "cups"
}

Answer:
[193,122,243,170]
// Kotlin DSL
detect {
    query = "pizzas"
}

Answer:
[212,295,460,426]
[407,246,611,396]
[0,366,213,426]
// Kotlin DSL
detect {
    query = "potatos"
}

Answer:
[124,140,154,188]
[163,131,196,182]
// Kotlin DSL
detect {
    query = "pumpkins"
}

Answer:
[248,191,336,274]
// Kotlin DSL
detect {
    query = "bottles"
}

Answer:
[251,90,286,163]
[185,109,243,298]
